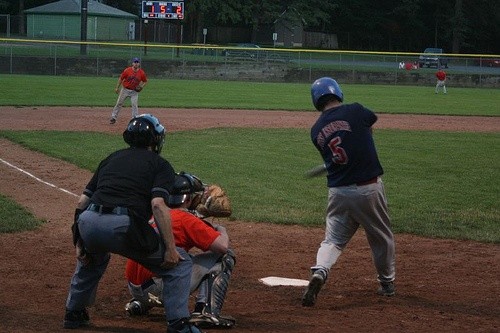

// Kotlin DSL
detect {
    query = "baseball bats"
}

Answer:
[305,164,328,180]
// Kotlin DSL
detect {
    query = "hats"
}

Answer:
[438,67,442,70]
[133,58,140,63]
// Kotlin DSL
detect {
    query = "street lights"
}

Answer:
[143,17,148,55]
[203,28,207,56]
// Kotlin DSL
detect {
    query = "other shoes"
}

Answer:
[110,119,115,124]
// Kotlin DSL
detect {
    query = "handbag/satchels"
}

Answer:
[127,210,159,254]
[72,202,92,246]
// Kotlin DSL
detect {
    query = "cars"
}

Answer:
[221,43,264,58]
[418,48,448,69]
[476,51,500,67]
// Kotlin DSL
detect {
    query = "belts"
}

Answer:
[357,176,377,186]
[128,279,154,291]
[87,203,129,215]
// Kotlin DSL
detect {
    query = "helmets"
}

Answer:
[167,174,192,207]
[126,118,161,145]
[311,77,343,111]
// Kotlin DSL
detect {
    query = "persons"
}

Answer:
[109,57,147,124]
[399,61,418,69]
[434,68,447,95]
[298,77,397,306]
[62,114,237,333]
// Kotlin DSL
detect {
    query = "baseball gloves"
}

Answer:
[190,183,231,217]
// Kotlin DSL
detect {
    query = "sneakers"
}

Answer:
[378,282,395,296]
[126,300,143,318]
[302,269,328,306]
[64,307,89,328]
[166,321,203,333]
[190,312,233,329]
